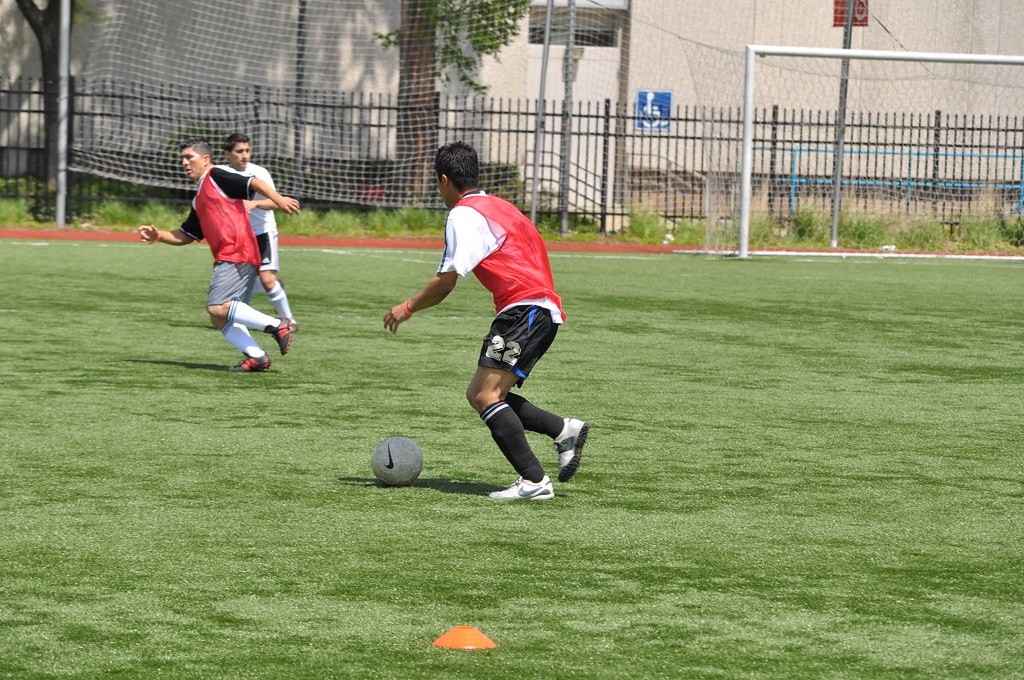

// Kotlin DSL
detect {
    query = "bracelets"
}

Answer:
[155,230,161,241]
[401,300,413,317]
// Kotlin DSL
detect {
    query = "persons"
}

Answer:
[139,139,302,372]
[223,133,298,331]
[382,141,589,502]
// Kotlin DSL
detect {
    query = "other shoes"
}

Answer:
[287,323,298,333]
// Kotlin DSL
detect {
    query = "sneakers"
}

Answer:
[264,317,293,355]
[554,417,588,484]
[489,474,554,500]
[228,352,272,373]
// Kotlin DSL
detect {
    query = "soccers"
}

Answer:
[371,435,424,488]
